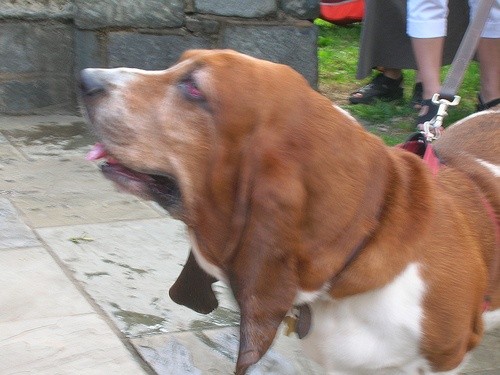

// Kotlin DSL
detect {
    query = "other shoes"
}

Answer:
[417,99,439,123]
[475,94,500,112]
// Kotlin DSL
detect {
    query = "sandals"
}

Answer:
[411,82,423,110]
[349,73,403,104]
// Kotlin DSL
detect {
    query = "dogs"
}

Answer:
[78,48,500,375]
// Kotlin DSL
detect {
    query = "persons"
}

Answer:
[349,0,480,110]
[406,0,500,132]
